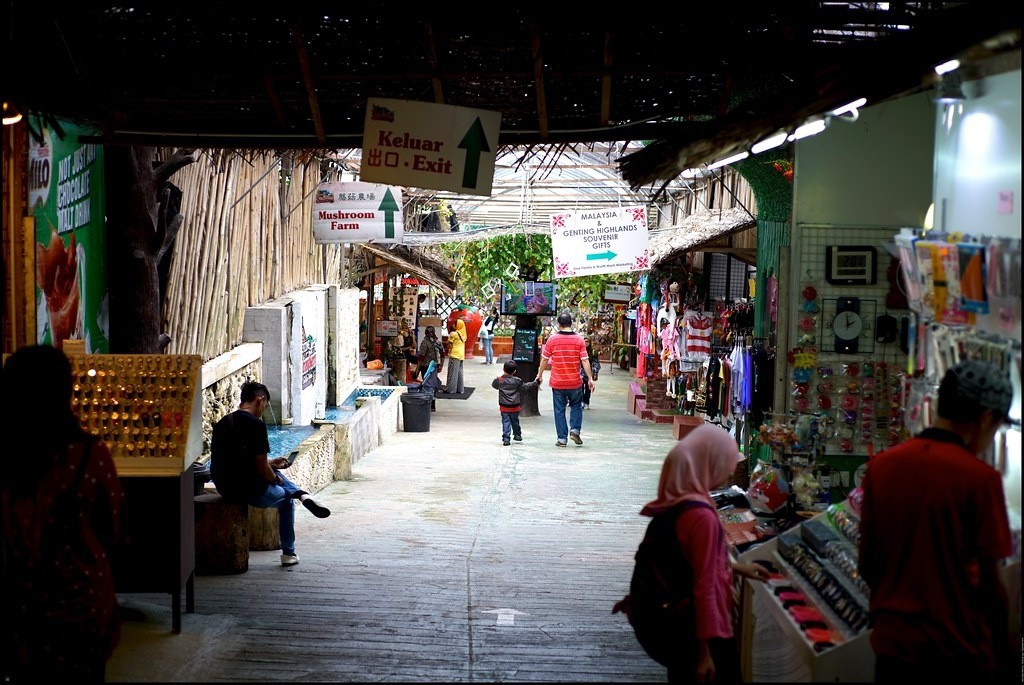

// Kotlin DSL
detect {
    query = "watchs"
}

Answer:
[826,488,872,597]
[69,355,192,456]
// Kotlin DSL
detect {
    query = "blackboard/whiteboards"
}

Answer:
[512,328,537,362]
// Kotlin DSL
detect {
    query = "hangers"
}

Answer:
[696,307,749,358]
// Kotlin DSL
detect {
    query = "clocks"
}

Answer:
[819,296,877,355]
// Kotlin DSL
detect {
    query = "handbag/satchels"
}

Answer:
[478,339,483,350]
[478,325,488,339]
[592,361,598,380]
[486,321,493,330]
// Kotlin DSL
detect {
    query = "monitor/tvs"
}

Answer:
[501,280,557,316]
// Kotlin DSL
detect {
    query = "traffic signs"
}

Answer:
[358,96,502,198]
[312,180,405,245]
[549,204,652,279]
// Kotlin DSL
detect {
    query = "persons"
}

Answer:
[441,318,467,394]
[416,326,445,412]
[482,307,499,364]
[565,337,600,410]
[628,424,771,685]
[0,342,126,685]
[858,361,1024,685]
[211,381,330,565]
[416,294,426,324]
[492,360,542,445]
[392,323,421,382]
[521,288,549,314]
[537,313,595,446]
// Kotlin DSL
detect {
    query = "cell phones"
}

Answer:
[286,451,299,466]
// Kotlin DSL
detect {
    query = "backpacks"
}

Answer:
[613,500,715,667]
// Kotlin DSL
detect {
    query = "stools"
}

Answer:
[194,494,281,575]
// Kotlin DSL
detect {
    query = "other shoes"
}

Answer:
[513,435,522,441]
[582,404,584,409]
[586,405,589,409]
[281,553,300,567]
[570,432,583,445]
[301,493,331,519]
[490,362,492,364]
[503,442,510,445]
[483,362,487,364]
[555,441,566,447]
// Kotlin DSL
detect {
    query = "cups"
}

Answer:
[47,243,86,351]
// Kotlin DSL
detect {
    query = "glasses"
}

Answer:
[585,341,588,342]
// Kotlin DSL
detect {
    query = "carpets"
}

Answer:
[436,386,475,400]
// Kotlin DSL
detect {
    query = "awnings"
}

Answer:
[352,241,458,297]
[620,1,1022,195]
[649,208,757,267]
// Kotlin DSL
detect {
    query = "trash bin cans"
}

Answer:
[401,392,432,432]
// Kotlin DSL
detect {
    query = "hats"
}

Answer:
[938,361,1019,429]
